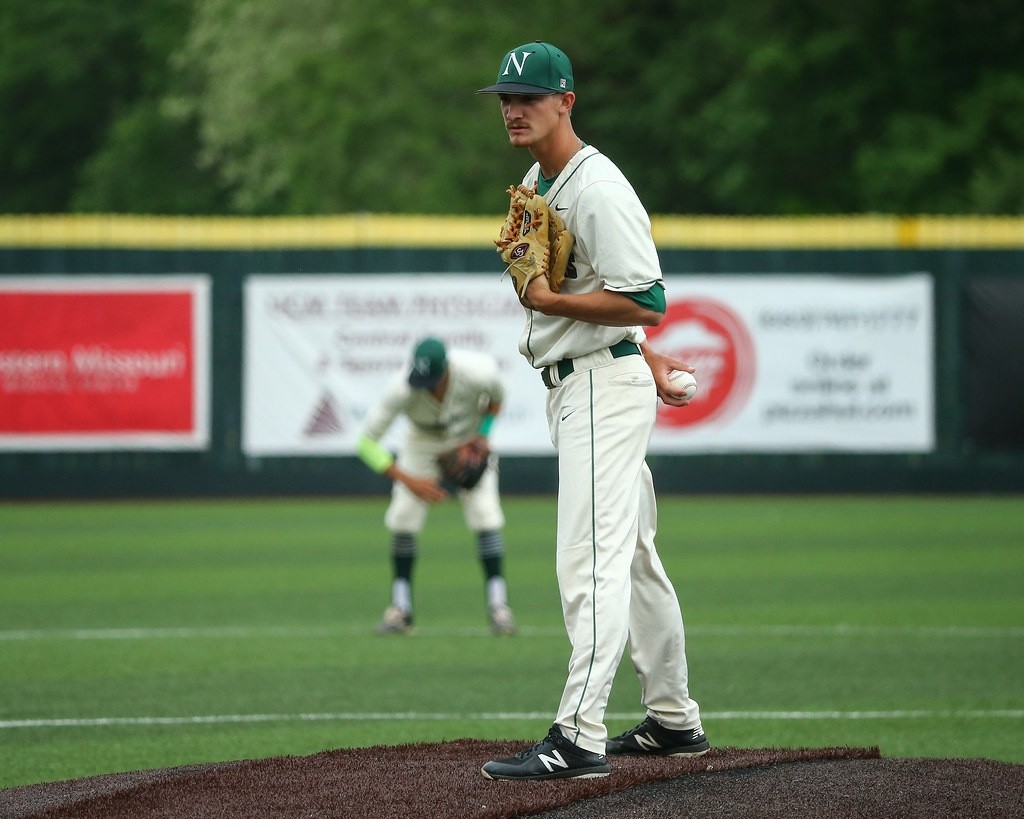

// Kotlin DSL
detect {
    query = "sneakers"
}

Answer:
[481,722,611,780]
[606,717,710,757]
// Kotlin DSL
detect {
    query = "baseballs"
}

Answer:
[668,371,698,400]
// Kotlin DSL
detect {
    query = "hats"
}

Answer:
[409,341,446,386]
[477,40,574,95]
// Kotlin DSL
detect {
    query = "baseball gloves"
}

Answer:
[495,183,574,301]
[432,444,493,492]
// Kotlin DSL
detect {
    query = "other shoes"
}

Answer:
[385,609,413,633]
[490,604,516,635]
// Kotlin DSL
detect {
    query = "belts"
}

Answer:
[541,341,640,388]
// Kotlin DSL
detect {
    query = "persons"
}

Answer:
[365,337,517,638]
[473,40,710,779]
